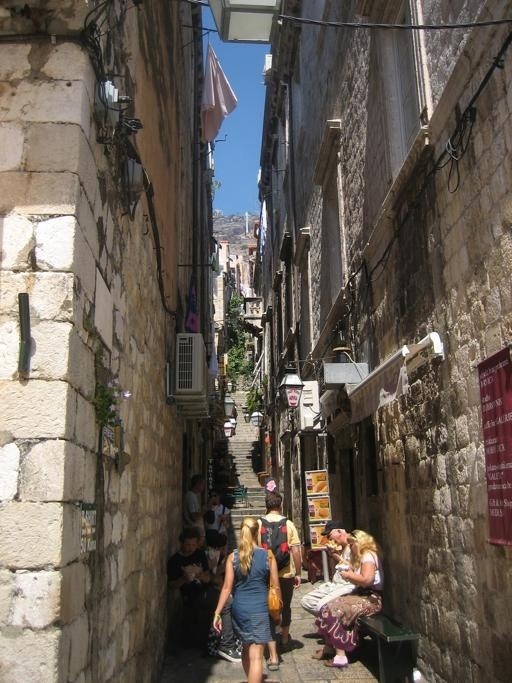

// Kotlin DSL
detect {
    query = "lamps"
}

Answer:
[252,411,266,427]
[277,356,335,409]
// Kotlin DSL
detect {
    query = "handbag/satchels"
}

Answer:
[205,529,220,545]
[266,584,284,626]
[359,569,382,590]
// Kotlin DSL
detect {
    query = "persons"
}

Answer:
[313,528,385,666]
[297,519,362,619]
[254,490,302,671]
[212,515,284,683]
[182,473,232,592]
[167,526,243,662]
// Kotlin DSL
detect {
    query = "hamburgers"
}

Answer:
[319,508,329,518]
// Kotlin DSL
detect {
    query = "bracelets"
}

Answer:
[215,612,221,618]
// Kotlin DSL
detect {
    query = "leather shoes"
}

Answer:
[312,649,349,668]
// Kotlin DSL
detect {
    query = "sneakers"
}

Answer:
[267,655,280,672]
[216,645,242,664]
[279,632,291,649]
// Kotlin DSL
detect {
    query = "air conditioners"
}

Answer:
[166,333,209,418]
[294,381,322,435]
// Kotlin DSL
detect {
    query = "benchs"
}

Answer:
[358,613,420,683]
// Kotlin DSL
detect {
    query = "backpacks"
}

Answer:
[259,517,290,573]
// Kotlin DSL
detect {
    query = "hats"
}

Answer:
[320,520,347,535]
